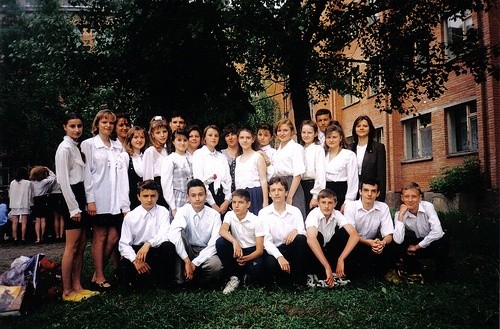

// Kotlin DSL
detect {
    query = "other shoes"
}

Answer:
[93,277,117,292]
[324,271,351,288]
[307,274,325,288]
[79,288,101,298]
[62,291,91,304]
[222,276,241,295]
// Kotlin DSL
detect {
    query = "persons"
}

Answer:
[80,108,123,291]
[395,182,453,284]
[8,167,34,245]
[257,176,309,288]
[117,179,180,295]
[213,190,266,293]
[168,179,224,290]
[48,179,67,239]
[340,178,393,284]
[112,109,387,218]
[53,115,101,302]
[29,165,57,245]
[304,189,359,288]
